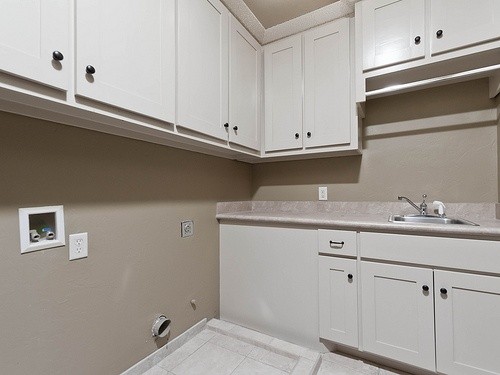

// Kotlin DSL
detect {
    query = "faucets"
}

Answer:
[398,194,428,216]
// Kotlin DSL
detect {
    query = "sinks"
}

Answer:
[387,214,480,227]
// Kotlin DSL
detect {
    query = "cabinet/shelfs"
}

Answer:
[322,228,360,348]
[264,16,365,152]
[353,0,500,103]
[0,0,175,127]
[177,0,263,152]
[360,231,500,374]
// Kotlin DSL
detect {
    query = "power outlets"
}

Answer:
[69,232,88,261]
[320,187,328,201]
[182,220,195,237]
